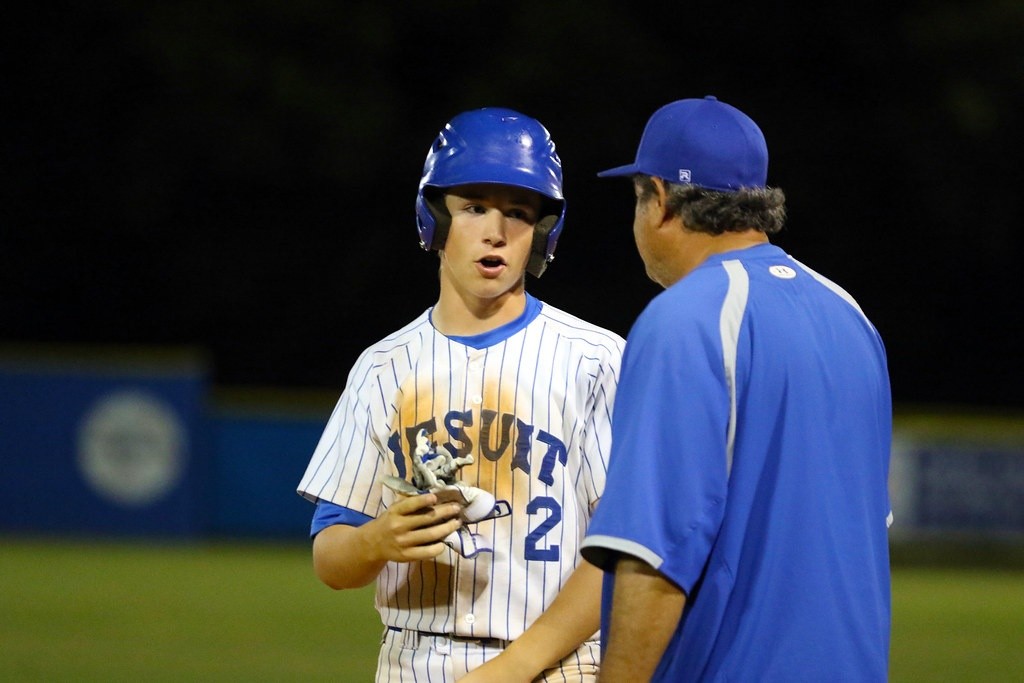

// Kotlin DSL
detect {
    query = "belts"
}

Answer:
[388,626,501,643]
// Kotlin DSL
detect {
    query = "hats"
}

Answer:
[597,95,769,194]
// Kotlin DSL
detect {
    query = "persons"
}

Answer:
[579,94,894,683]
[298,109,628,683]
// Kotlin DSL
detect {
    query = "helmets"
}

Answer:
[416,107,566,279]
